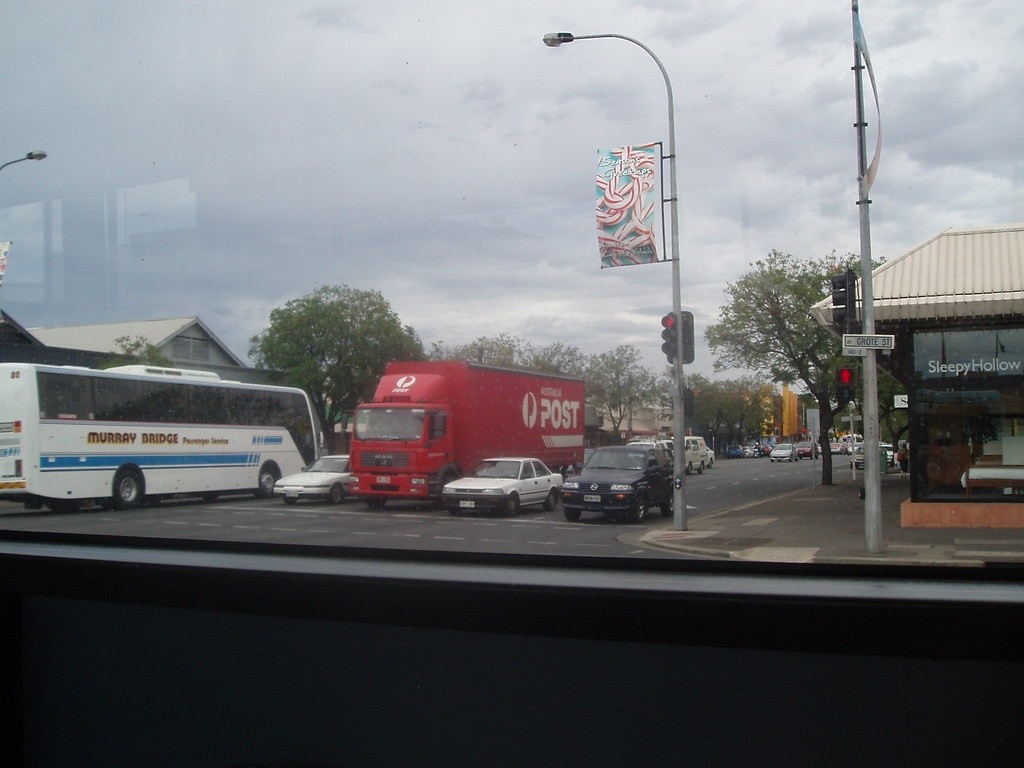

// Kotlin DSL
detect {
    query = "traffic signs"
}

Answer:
[843,333,896,351]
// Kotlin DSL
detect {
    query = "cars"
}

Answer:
[849,445,888,468]
[440,456,564,514]
[879,445,894,464]
[623,433,714,479]
[829,434,866,455]
[817,444,823,454]
[273,454,353,506]
[769,443,800,462]
[726,444,746,458]
[796,441,819,459]
[742,445,774,457]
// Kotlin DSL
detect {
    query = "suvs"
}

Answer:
[563,445,677,522]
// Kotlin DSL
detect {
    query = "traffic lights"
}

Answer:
[829,271,860,330]
[836,365,858,387]
[660,310,678,365]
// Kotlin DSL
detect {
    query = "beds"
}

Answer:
[960,454,1024,499]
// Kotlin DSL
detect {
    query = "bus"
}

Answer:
[0,363,327,509]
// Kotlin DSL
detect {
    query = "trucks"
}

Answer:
[340,359,586,510]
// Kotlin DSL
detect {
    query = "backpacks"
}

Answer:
[897,451,905,462]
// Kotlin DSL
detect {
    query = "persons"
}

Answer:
[898,443,910,480]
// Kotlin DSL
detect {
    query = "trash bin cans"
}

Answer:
[879,447,888,475]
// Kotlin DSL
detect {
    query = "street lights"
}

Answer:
[542,28,688,528]
